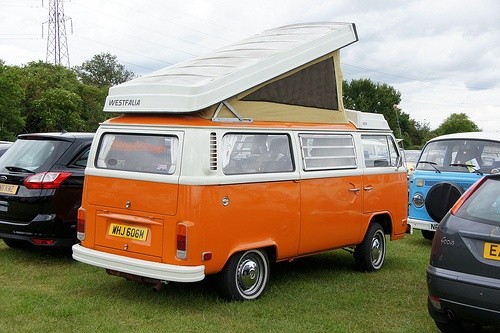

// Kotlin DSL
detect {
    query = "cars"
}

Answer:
[426,172,500,333]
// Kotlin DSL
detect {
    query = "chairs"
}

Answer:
[264,139,293,172]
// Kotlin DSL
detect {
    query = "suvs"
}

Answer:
[0,132,96,261]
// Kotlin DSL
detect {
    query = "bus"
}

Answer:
[406,132,500,240]
[71,95,409,302]
